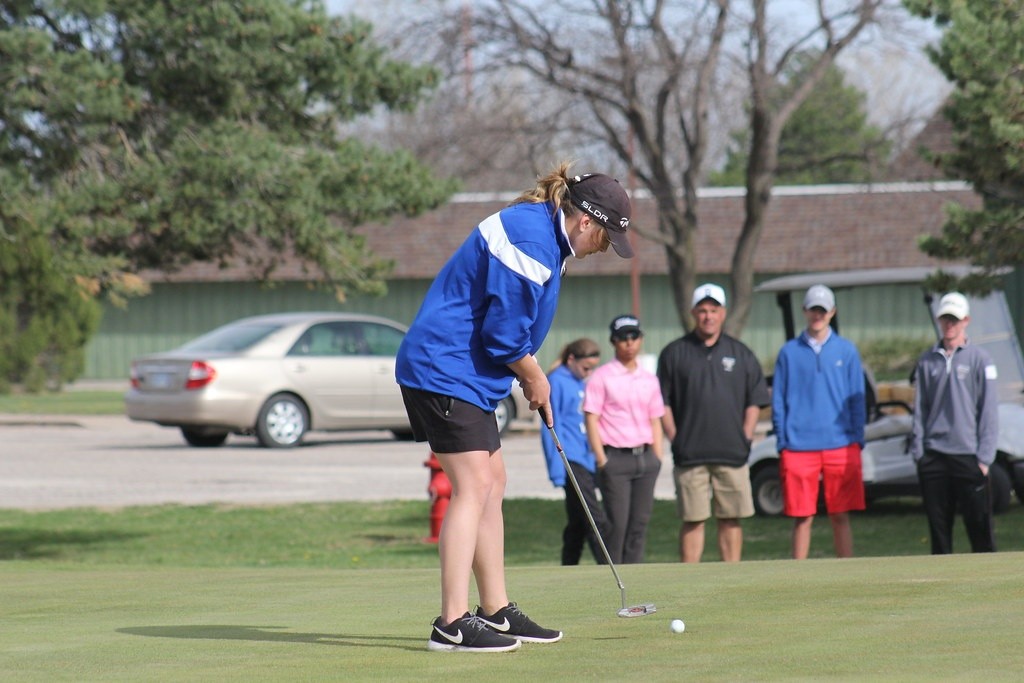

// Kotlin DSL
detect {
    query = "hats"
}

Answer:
[804,284,835,312]
[691,284,725,309]
[610,316,640,335]
[565,173,634,259]
[936,293,968,320]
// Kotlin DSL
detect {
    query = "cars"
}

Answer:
[129,312,535,449]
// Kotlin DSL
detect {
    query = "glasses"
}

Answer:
[619,333,638,341]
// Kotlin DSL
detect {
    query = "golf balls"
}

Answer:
[669,619,686,635]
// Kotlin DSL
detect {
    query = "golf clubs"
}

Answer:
[537,407,656,618]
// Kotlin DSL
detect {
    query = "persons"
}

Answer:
[541,314,665,565]
[656,283,771,563]
[771,284,867,559]
[395,157,635,653]
[909,292,999,555]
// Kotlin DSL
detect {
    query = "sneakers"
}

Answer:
[476,601,562,643]
[427,611,522,652]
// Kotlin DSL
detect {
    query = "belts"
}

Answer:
[622,444,648,454]
